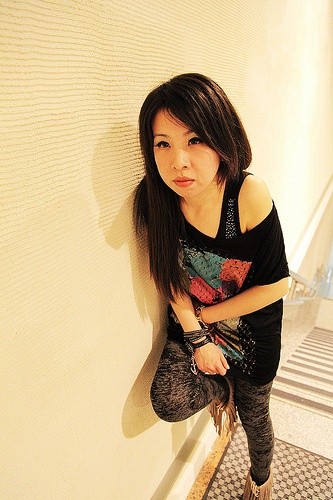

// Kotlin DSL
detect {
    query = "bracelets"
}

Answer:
[193,304,209,331]
[184,329,209,342]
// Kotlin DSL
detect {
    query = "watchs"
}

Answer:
[184,338,211,354]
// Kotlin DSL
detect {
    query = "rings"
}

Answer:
[211,372,216,375]
[205,372,210,375]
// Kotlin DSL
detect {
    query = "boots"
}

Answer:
[243,468,273,500]
[208,372,238,437]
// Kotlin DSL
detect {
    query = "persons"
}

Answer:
[128,72,292,500]
